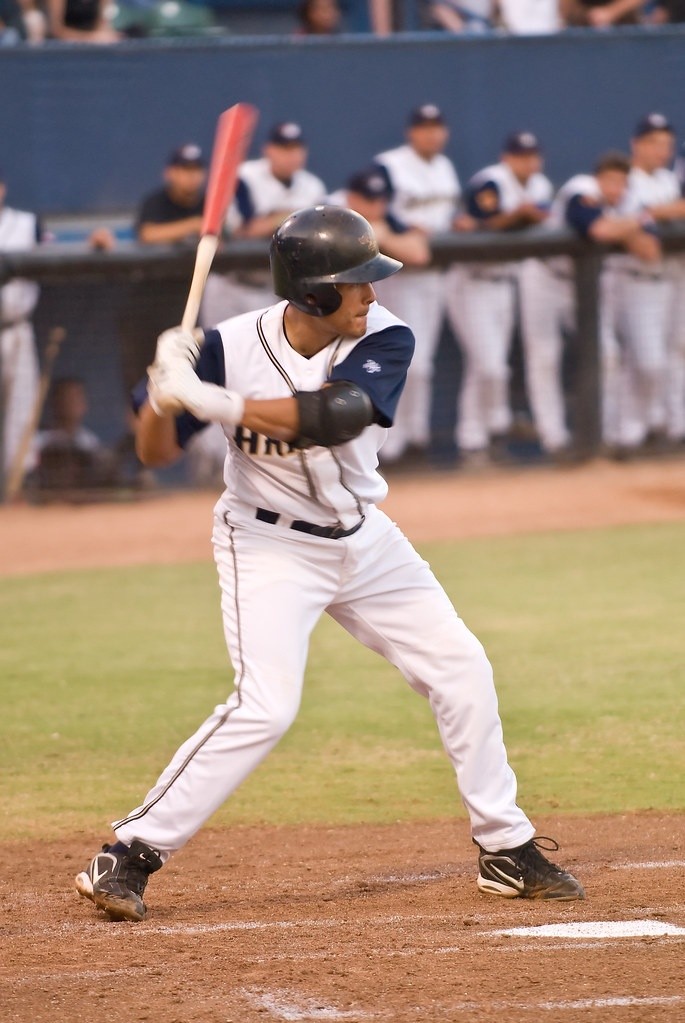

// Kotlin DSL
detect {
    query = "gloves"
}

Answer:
[151,358,244,426]
[151,325,204,367]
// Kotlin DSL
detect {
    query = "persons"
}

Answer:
[0,0,127,46]
[26,377,151,486]
[126,104,685,465]
[73,205,586,922]
[295,0,685,37]
[0,180,55,493]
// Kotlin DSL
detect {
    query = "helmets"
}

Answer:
[270,205,403,316]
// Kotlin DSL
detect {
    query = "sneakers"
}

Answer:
[75,839,163,921]
[472,837,586,901]
[378,429,684,475]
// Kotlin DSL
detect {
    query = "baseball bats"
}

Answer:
[158,100,261,415]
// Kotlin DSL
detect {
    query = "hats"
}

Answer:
[263,119,309,145]
[348,162,395,197]
[410,102,445,125]
[165,143,209,166]
[634,112,676,137]
[506,132,540,154]
